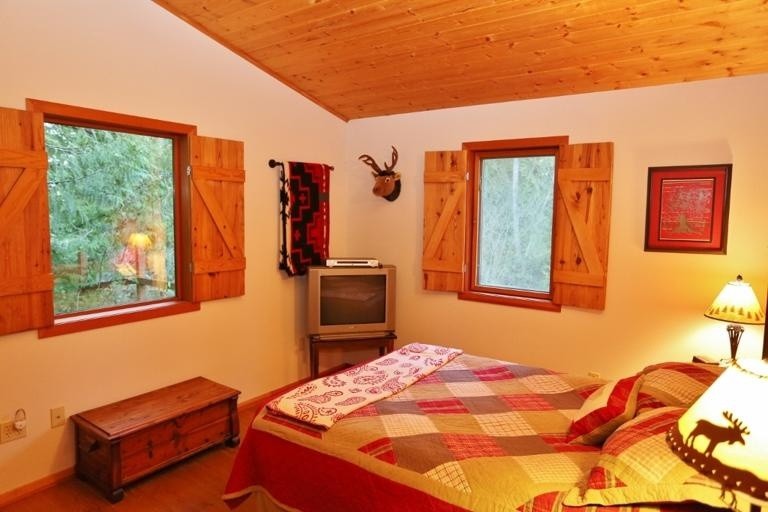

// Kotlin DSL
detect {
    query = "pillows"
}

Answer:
[559,356,742,512]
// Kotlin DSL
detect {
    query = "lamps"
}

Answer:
[664,360,767,503]
[704,273,766,361]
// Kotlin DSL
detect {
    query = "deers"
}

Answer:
[358,145,403,202]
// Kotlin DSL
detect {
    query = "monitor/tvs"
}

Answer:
[306,265,397,340]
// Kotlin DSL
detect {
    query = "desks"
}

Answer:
[306,333,399,382]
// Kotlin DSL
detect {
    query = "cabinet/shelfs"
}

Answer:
[66,376,242,505]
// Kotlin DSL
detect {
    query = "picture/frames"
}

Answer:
[643,162,731,257]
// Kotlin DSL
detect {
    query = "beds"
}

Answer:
[251,335,768,512]
